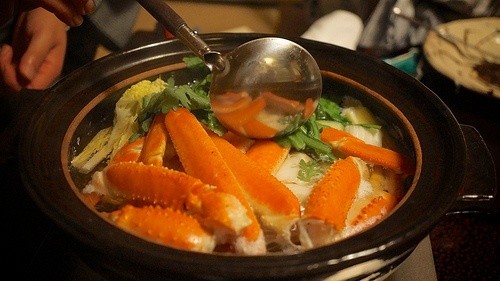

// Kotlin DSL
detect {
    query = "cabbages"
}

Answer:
[70,78,170,175]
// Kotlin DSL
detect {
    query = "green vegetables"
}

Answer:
[129,55,382,181]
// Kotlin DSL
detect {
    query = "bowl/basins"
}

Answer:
[23,29,488,281]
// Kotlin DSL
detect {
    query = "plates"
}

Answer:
[427,17,499,99]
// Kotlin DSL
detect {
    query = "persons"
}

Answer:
[0,1,140,95]
